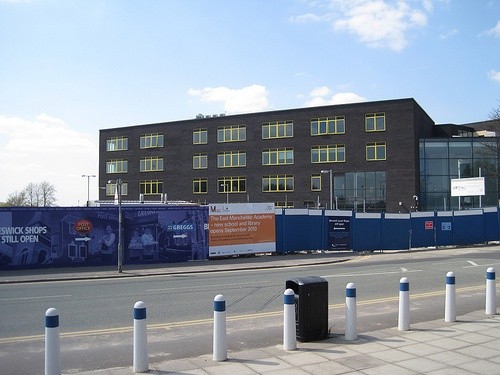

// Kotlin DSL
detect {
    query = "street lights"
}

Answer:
[82,175,97,207]
[321,169,334,209]
[108,178,124,273]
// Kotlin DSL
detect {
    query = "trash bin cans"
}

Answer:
[285,275,330,342]
